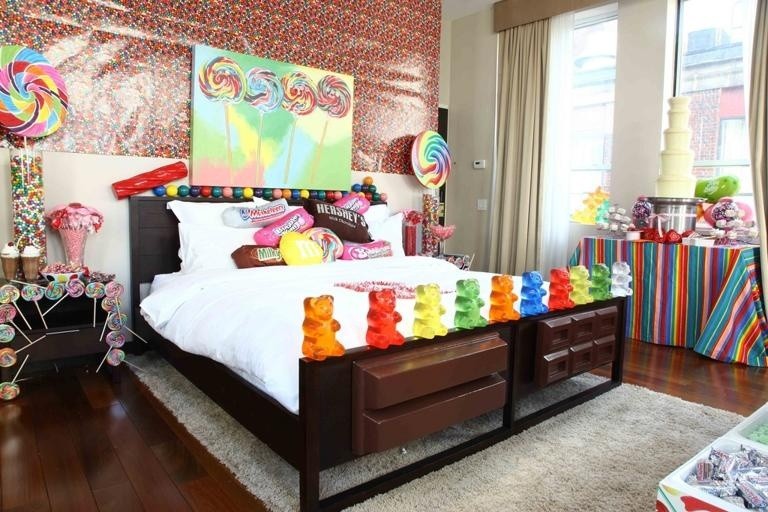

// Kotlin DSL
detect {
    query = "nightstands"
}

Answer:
[438,254,471,271]
[0,274,121,394]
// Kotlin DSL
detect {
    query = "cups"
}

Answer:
[421,188,440,256]
[0,255,20,284]
[9,148,47,276]
[622,230,644,240]
[402,219,416,256]
[20,256,41,283]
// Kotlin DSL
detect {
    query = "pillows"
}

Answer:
[163,199,406,274]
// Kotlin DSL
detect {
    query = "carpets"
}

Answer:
[122,350,742,511]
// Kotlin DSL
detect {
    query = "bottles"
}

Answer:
[632,197,652,229]
[711,198,739,225]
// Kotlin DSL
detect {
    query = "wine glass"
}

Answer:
[429,226,456,260]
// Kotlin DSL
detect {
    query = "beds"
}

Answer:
[128,193,637,511]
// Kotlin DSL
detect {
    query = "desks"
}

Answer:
[567,233,767,367]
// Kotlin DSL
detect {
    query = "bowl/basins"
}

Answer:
[41,271,84,284]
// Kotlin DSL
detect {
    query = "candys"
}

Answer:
[198,56,351,187]
[593,195,760,242]
[689,418,768,512]
[0,44,126,284]
[1,278,128,403]
[409,130,472,272]
